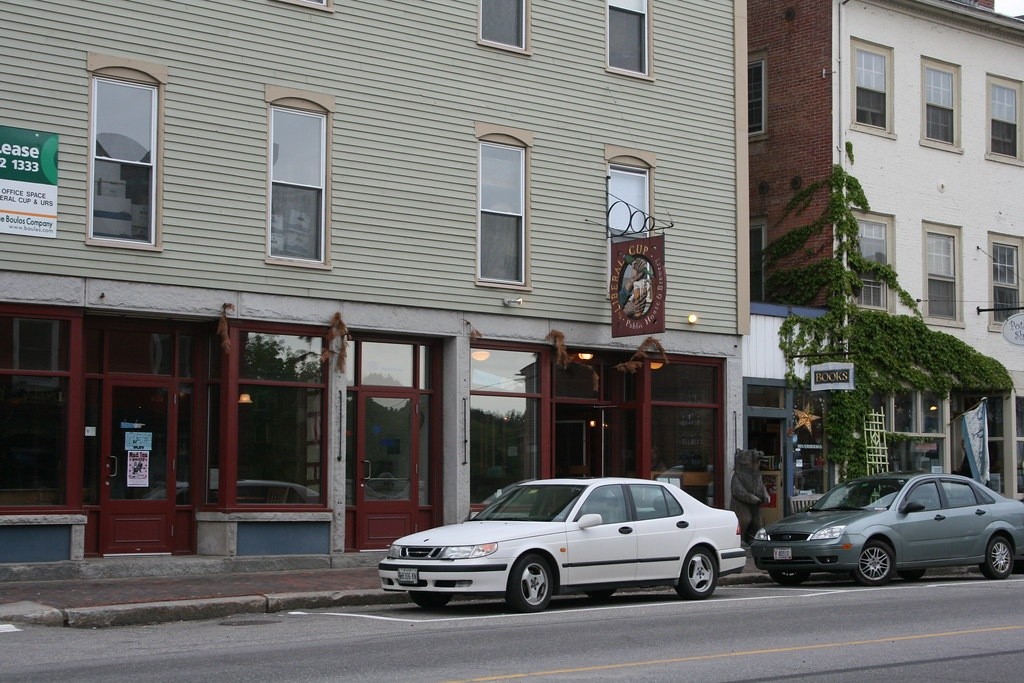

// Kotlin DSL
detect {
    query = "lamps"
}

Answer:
[503,295,523,306]
[688,314,698,325]
[238,394,253,403]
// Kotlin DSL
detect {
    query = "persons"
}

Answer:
[651,446,668,471]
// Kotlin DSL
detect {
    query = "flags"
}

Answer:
[962,401,990,487]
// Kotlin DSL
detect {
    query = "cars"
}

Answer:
[233,479,320,504]
[377,476,748,614]
[750,471,1024,585]
[144,480,218,508]
